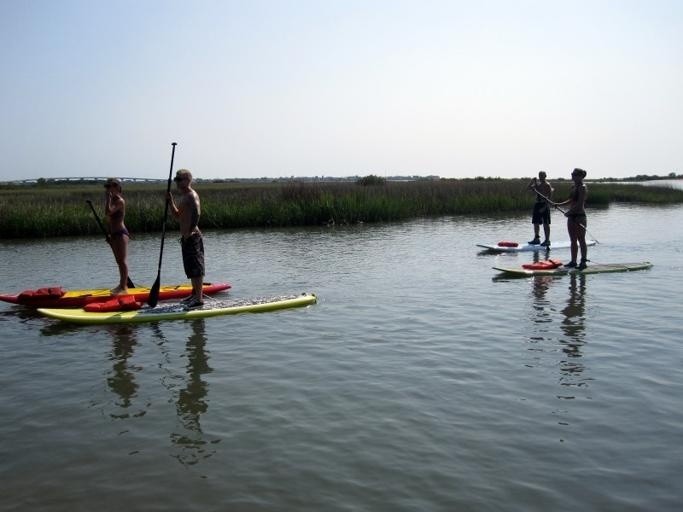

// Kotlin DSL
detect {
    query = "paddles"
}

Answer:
[87,199,135,289]
[148,142,176,308]
[532,185,598,244]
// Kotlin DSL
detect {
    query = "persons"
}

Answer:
[527,171,552,246]
[102,178,131,294]
[553,168,587,270]
[167,169,206,310]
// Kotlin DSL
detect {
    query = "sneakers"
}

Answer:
[564,261,587,268]
[109,285,128,295]
[528,239,540,244]
[181,295,204,310]
[541,240,550,245]
